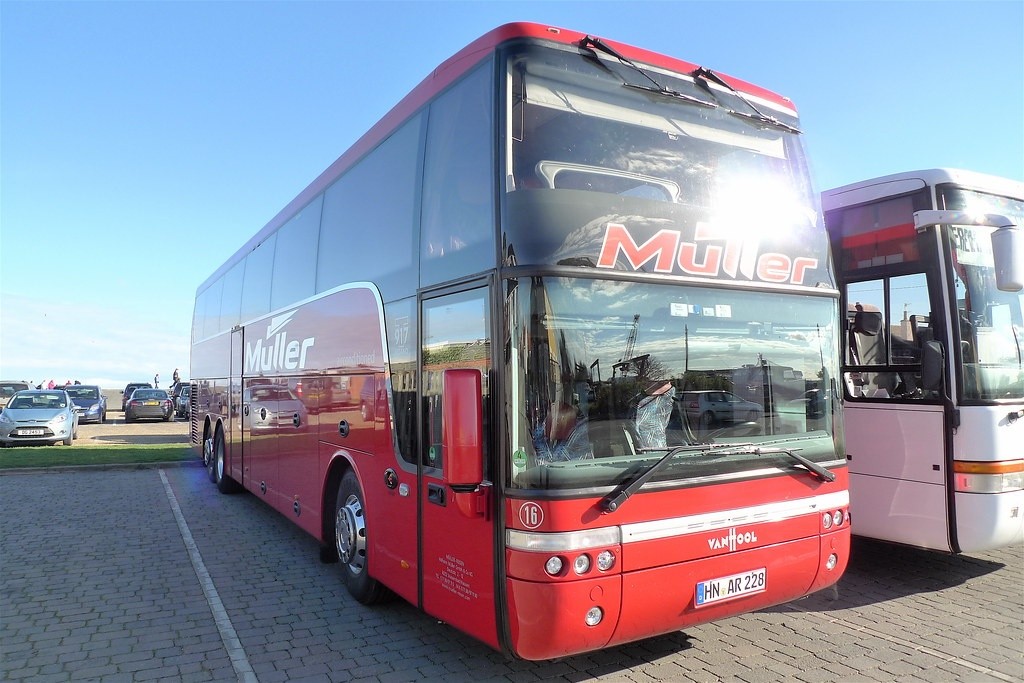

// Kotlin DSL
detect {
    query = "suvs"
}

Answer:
[0,380,45,413]
[120,383,154,412]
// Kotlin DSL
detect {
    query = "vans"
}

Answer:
[242,363,387,425]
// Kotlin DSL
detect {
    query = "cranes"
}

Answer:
[616,312,653,379]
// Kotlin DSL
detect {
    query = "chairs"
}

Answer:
[68,391,78,397]
[527,399,578,448]
[849,303,902,394]
[910,315,934,343]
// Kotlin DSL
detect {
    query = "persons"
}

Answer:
[66,380,81,386]
[218,402,223,415]
[206,400,210,410]
[154,373,159,389]
[40,379,54,390]
[233,402,236,414]
[625,336,676,456]
[169,368,179,389]
[533,398,583,467]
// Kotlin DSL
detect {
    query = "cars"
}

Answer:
[58,385,108,424]
[170,381,191,420]
[238,384,309,434]
[790,388,829,418]
[0,390,82,447]
[676,391,763,426]
[124,389,176,423]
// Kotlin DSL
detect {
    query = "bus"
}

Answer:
[823,169,1023,553]
[183,20,851,672]
